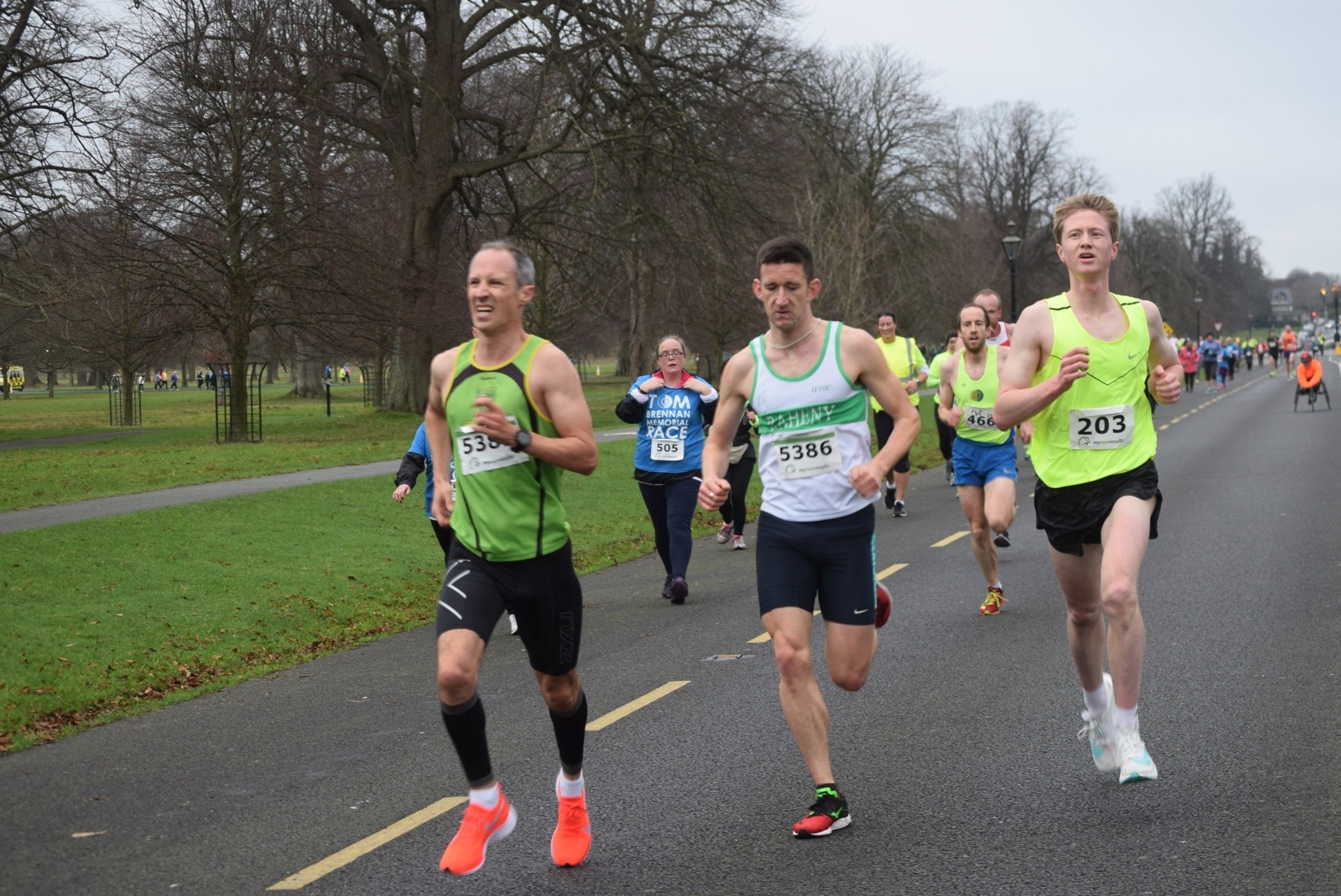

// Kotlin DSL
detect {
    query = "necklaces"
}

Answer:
[765,316,821,350]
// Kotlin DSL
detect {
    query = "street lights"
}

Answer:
[1000,219,1024,321]
[1320,282,1341,333]
[1193,290,1205,346]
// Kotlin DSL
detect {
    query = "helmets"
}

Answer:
[1300,352,1313,363]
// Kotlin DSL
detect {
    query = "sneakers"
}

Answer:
[994,531,1011,548]
[672,577,688,604]
[945,461,953,481]
[949,472,957,485]
[892,501,907,518]
[716,521,735,544]
[873,580,894,628]
[439,787,520,875]
[510,615,521,634]
[884,484,898,509]
[663,575,673,597]
[1082,671,1123,774]
[979,584,1005,615]
[550,775,591,866]
[793,793,851,839]
[732,534,746,549]
[1023,443,1032,461]
[1114,726,1158,782]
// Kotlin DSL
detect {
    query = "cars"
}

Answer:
[1298,316,1337,351]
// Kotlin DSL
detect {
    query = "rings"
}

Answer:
[687,383,690,385]
[707,492,715,499]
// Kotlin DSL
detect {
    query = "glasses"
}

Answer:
[659,350,686,359]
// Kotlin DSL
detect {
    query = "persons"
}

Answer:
[937,302,1034,616]
[926,332,964,489]
[614,335,725,604]
[392,412,521,638]
[421,240,603,878]
[701,352,761,551]
[1143,318,1332,418]
[104,358,353,396]
[950,287,1034,548]
[696,238,923,844]
[864,309,930,517]
[992,193,1185,788]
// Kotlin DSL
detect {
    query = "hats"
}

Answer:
[1206,332,1213,336]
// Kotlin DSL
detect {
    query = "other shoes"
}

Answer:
[1185,362,1296,395]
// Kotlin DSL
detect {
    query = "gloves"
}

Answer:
[1204,348,1210,354]
[1208,349,1214,356]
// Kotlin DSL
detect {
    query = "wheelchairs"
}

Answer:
[1294,380,1332,413]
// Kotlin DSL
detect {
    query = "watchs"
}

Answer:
[509,427,532,454]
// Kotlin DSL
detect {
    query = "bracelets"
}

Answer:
[637,386,650,396]
[915,379,922,387]
